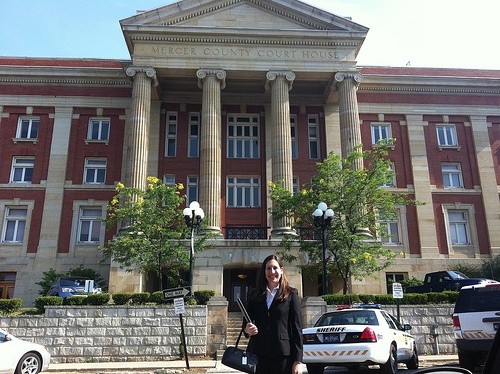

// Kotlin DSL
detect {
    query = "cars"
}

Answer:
[301,304,420,374]
[0,329,51,374]
[45,277,97,299]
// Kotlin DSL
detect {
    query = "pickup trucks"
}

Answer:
[405,270,499,293]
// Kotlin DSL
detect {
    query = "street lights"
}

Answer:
[313,202,334,295]
[183,200,205,286]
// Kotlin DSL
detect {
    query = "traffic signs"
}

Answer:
[162,286,193,300]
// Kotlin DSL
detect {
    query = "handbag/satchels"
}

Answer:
[221,324,259,374]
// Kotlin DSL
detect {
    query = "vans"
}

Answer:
[451,283,500,369]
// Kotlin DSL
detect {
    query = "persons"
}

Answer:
[242,255,303,374]
